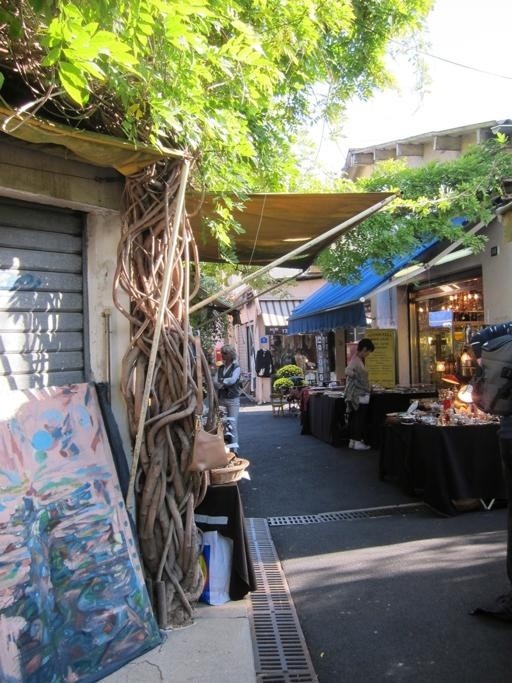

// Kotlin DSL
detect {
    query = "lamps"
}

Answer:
[441,372,482,412]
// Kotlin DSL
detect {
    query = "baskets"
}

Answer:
[210,458,251,484]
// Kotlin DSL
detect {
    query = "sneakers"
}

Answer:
[470,592,512,621]
[348,438,372,451]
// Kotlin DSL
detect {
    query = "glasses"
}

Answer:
[222,352,229,356]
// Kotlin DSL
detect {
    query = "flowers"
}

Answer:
[273,377,294,389]
[277,364,304,376]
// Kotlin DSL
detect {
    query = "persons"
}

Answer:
[345,339,373,450]
[255,336,273,405]
[213,345,240,454]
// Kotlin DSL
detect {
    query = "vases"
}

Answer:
[280,389,287,395]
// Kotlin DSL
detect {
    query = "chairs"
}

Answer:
[270,393,286,417]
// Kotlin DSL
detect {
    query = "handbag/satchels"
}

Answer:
[185,419,236,473]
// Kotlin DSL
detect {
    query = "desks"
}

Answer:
[378,423,502,499]
[301,392,437,447]
